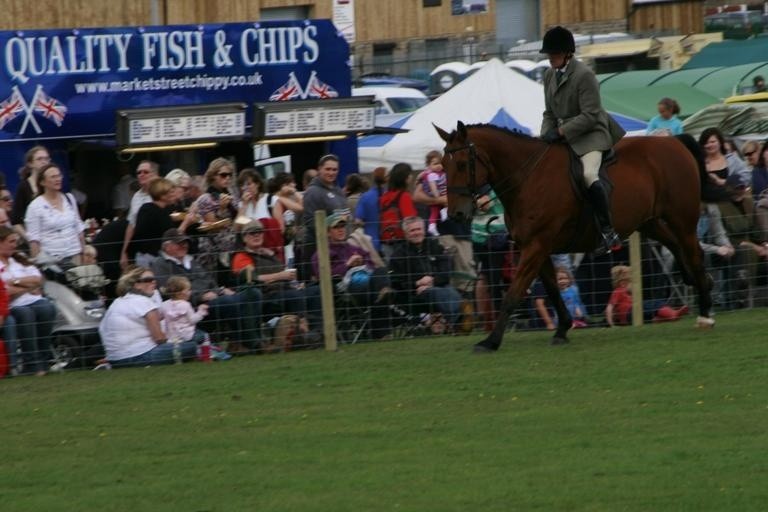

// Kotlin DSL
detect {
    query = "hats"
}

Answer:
[162,229,192,243]
[242,223,260,237]
[324,215,344,228]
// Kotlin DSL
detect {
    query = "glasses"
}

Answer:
[217,172,232,178]
[139,276,155,283]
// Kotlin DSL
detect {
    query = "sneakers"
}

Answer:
[376,289,394,305]
[429,224,438,235]
[210,350,232,360]
[441,207,447,220]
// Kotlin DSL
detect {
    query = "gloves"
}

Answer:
[545,128,563,141]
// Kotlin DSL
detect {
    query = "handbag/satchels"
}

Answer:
[68,263,110,289]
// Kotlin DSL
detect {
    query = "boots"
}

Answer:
[659,306,687,320]
[586,181,619,258]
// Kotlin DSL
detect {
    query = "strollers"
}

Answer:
[36,263,108,368]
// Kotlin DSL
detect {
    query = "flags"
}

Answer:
[33,91,68,127]
[268,79,299,102]
[308,77,339,99]
[0,94,25,129]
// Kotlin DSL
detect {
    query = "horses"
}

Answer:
[431,121,737,352]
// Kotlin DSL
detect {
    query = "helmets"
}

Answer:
[540,27,575,53]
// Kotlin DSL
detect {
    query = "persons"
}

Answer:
[539,25,626,248]
[646,99,684,136]
[743,76,766,94]
[3,131,768,376]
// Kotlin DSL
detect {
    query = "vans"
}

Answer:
[703,10,764,41]
[504,31,634,62]
[349,84,430,134]
